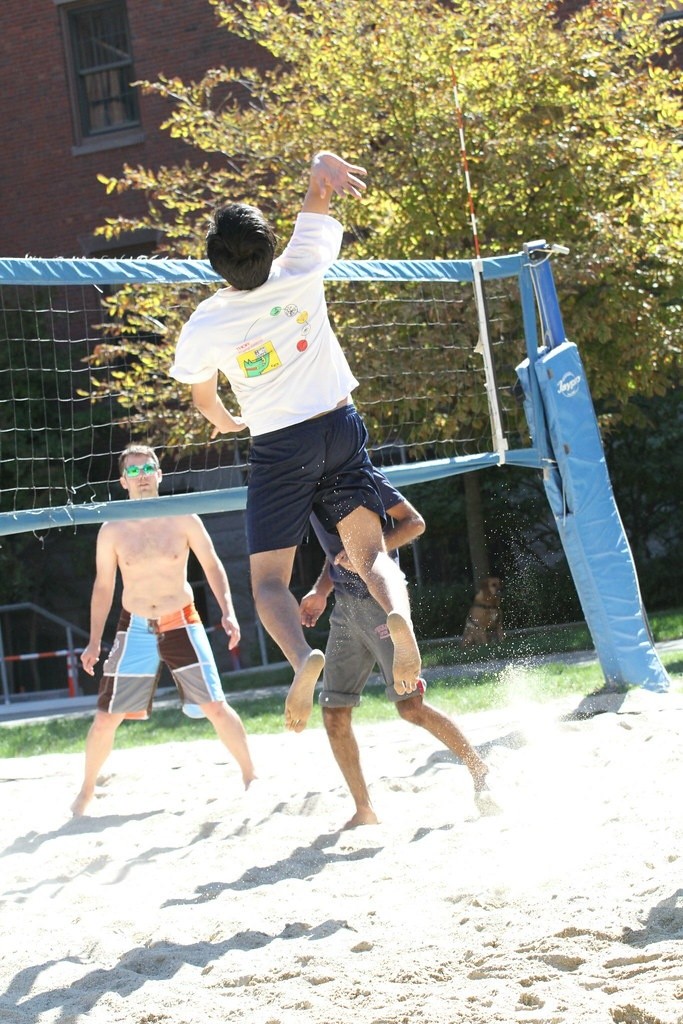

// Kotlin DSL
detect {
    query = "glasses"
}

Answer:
[124,465,157,478]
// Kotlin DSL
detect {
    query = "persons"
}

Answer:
[69,445,256,816]
[298,467,490,829]
[169,152,421,732]
